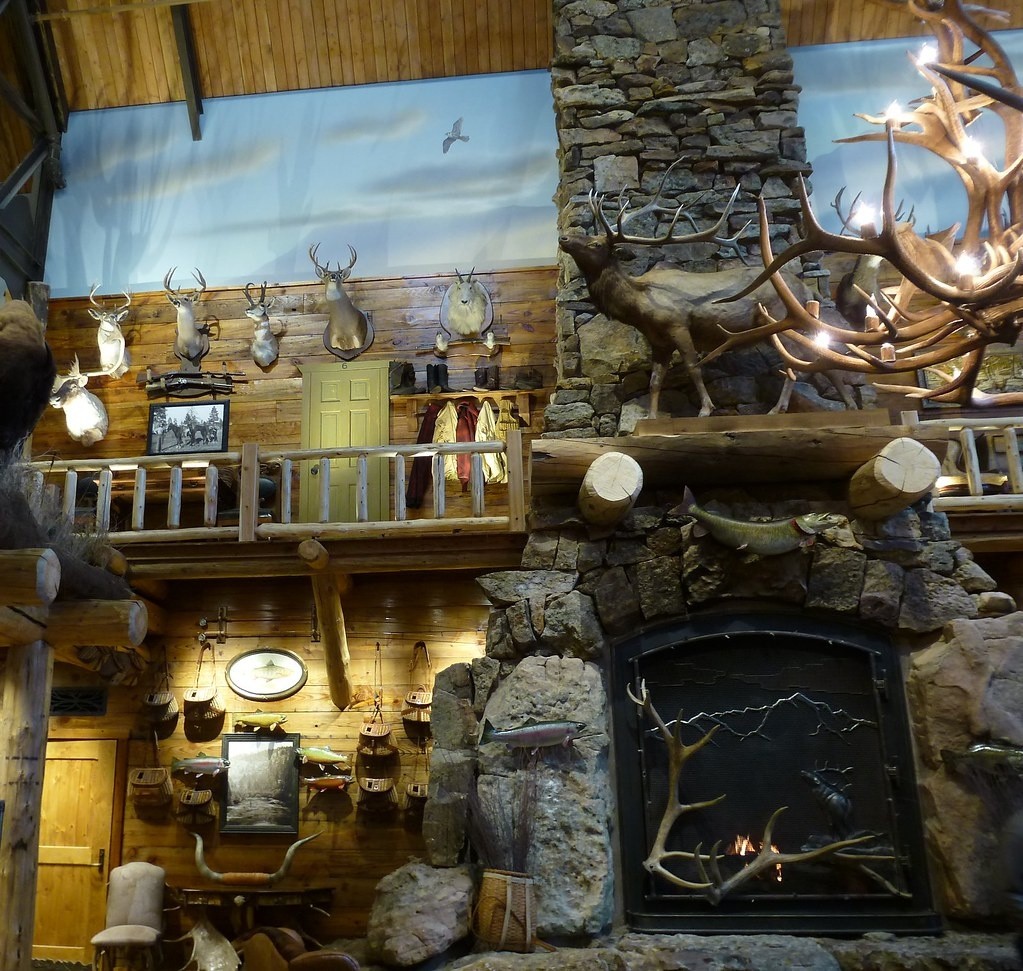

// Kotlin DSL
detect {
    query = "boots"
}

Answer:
[472,365,499,392]
[500,372,529,390]
[389,361,415,395]
[426,364,442,393]
[463,368,487,392]
[437,363,459,392]
[403,361,426,392]
[516,368,543,390]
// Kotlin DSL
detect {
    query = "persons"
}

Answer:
[181,419,216,445]
[154,423,168,453]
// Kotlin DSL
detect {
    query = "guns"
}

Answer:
[136,371,246,392]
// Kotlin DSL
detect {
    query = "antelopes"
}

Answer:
[447,266,487,335]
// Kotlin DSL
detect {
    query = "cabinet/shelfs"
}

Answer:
[975,432,1023,494]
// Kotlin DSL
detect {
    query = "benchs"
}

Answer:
[76,457,283,532]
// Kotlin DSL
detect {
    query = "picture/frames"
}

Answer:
[224,646,309,702]
[145,398,230,457]
[217,733,300,836]
[912,348,1023,411]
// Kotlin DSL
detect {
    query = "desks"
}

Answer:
[181,886,337,936]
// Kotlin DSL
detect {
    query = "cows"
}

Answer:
[47,339,125,447]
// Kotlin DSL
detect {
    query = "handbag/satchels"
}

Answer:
[182,642,226,723]
[357,642,398,758]
[141,644,179,730]
[126,729,174,808]
[175,753,216,825]
[470,868,536,952]
[402,724,428,818]
[400,642,434,722]
[357,759,398,814]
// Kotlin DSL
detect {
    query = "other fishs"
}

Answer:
[233,709,287,732]
[293,746,347,771]
[251,660,291,681]
[478,717,587,754]
[171,752,230,778]
[939,743,1023,779]
[299,771,355,793]
[801,770,848,812]
[668,485,839,557]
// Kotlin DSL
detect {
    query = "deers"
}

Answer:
[242,281,279,367]
[309,242,367,351]
[87,284,131,379]
[558,154,858,419]
[830,186,916,323]
[163,266,206,360]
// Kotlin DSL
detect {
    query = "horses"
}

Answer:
[187,423,210,445]
[167,423,191,445]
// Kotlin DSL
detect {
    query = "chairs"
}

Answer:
[90,861,166,971]
